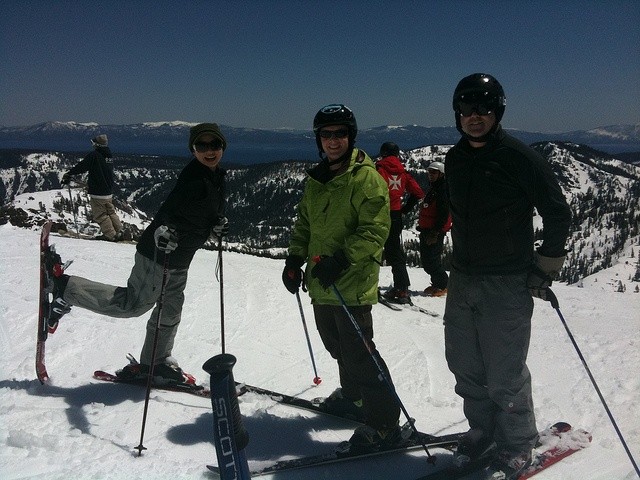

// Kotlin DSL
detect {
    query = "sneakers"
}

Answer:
[312,388,360,420]
[452,431,496,468]
[485,447,533,480]
[334,423,403,459]
[385,288,409,304]
[423,285,444,296]
[134,360,195,386]
[47,274,72,327]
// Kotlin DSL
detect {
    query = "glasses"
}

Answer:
[317,128,350,138]
[193,140,223,151]
[89,139,95,146]
[459,101,500,116]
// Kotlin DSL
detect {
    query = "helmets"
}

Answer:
[313,104,358,166]
[451,73,507,142]
[427,161,444,176]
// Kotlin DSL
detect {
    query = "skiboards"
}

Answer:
[411,421,593,479]
[36,219,247,398]
[379,286,449,297]
[206,380,470,476]
[57,229,141,244]
[376,292,441,317]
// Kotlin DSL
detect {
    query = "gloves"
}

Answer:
[154,225,178,254]
[311,249,350,289]
[213,217,230,238]
[282,254,307,293]
[62,170,75,185]
[528,269,557,300]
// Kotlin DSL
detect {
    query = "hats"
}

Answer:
[94,134,108,146]
[189,122,226,153]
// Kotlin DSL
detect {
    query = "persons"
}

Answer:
[441,72,571,480]
[63,134,125,242]
[375,142,425,304]
[418,162,451,296]
[282,103,404,452]
[47,123,226,385]
